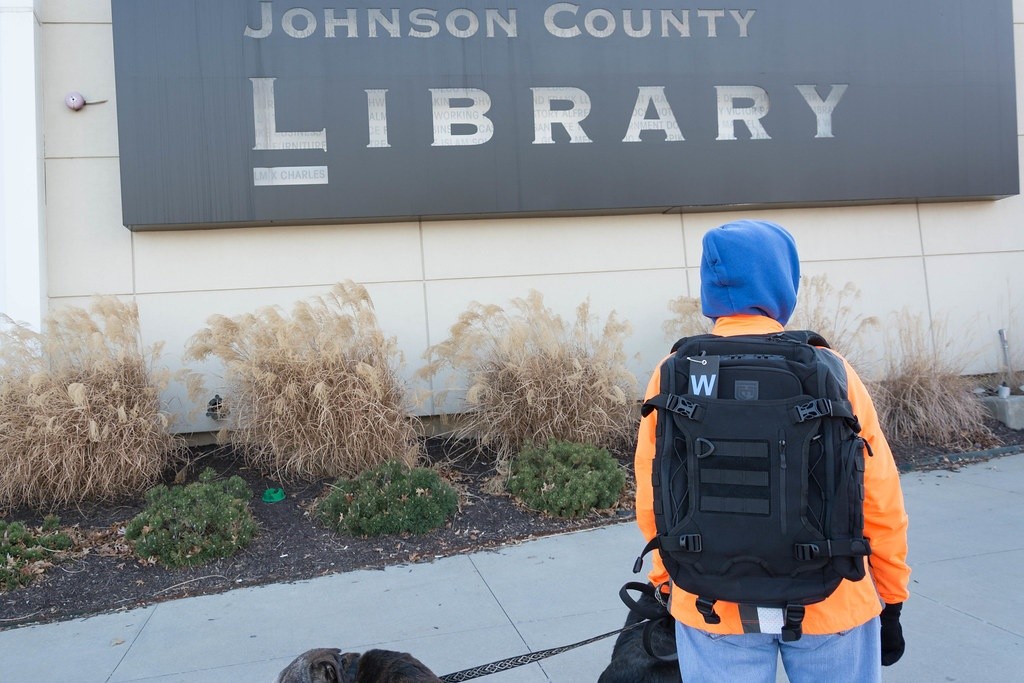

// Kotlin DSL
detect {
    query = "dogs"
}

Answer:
[271,647,443,683]
[598,573,682,683]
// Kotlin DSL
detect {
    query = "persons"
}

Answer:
[634,219,907,683]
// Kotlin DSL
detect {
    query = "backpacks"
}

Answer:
[650,331,866,609]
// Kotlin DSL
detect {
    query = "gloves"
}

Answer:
[880,601,906,667]
